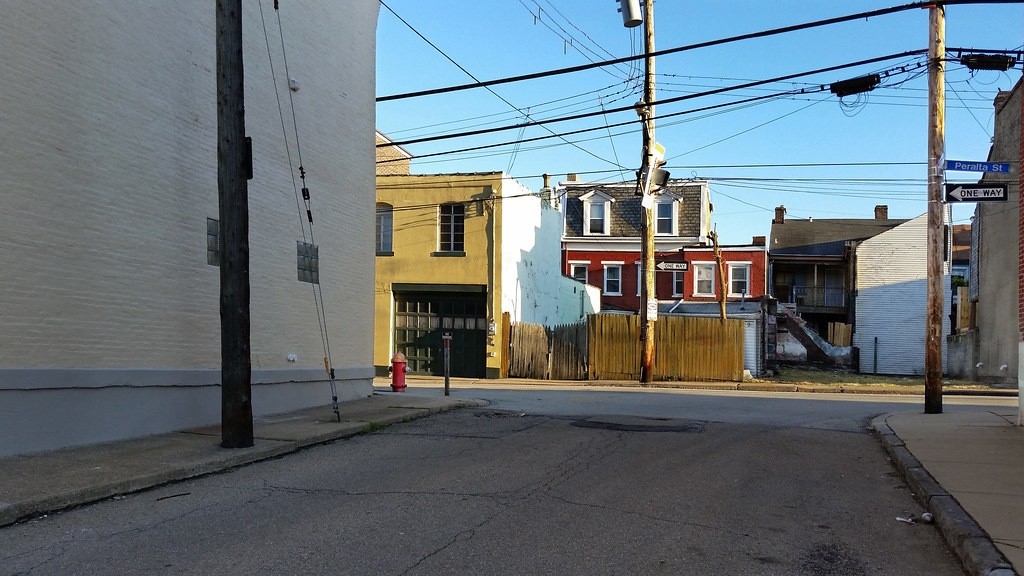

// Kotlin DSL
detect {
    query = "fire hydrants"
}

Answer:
[389,351,411,393]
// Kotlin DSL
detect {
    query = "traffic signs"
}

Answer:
[944,160,1009,173]
[655,260,689,272]
[945,183,1008,203]
